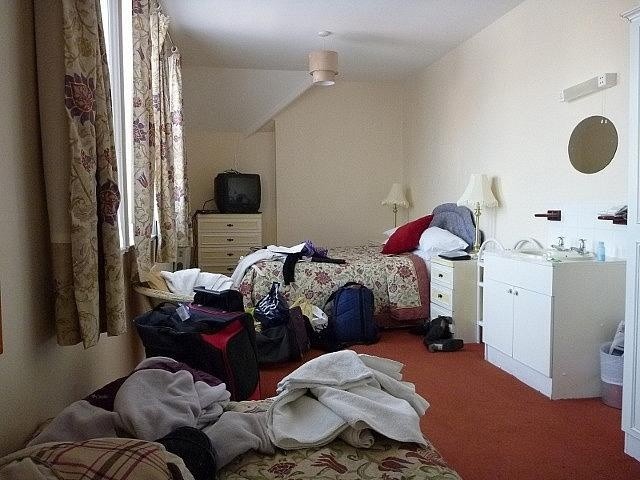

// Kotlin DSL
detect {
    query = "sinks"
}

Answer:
[519,246,591,261]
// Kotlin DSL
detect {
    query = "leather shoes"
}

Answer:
[408,321,431,336]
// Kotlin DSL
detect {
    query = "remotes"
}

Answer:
[200,210,206,213]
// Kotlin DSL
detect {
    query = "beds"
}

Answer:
[1,394,464,480]
[239,203,481,329]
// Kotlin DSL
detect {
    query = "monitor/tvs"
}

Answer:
[213,173,261,213]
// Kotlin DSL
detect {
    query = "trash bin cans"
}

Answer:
[600,342,624,408]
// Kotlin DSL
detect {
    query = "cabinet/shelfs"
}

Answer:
[197,211,263,277]
[480,250,625,399]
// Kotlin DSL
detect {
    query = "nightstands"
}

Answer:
[430,253,483,343]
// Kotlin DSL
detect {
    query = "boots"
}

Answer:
[425,336,464,353]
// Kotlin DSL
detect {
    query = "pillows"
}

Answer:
[383,214,433,254]
[416,225,468,259]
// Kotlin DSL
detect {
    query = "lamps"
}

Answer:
[458,174,499,258]
[309,31,338,86]
[382,182,409,229]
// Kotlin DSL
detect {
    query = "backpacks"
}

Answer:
[322,281,381,345]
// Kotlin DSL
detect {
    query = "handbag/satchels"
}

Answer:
[132,298,199,360]
[253,281,288,324]
[255,325,292,367]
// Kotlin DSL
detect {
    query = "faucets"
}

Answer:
[552,237,566,252]
[572,238,586,255]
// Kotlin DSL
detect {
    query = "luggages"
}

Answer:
[200,319,260,402]
[287,305,312,359]
